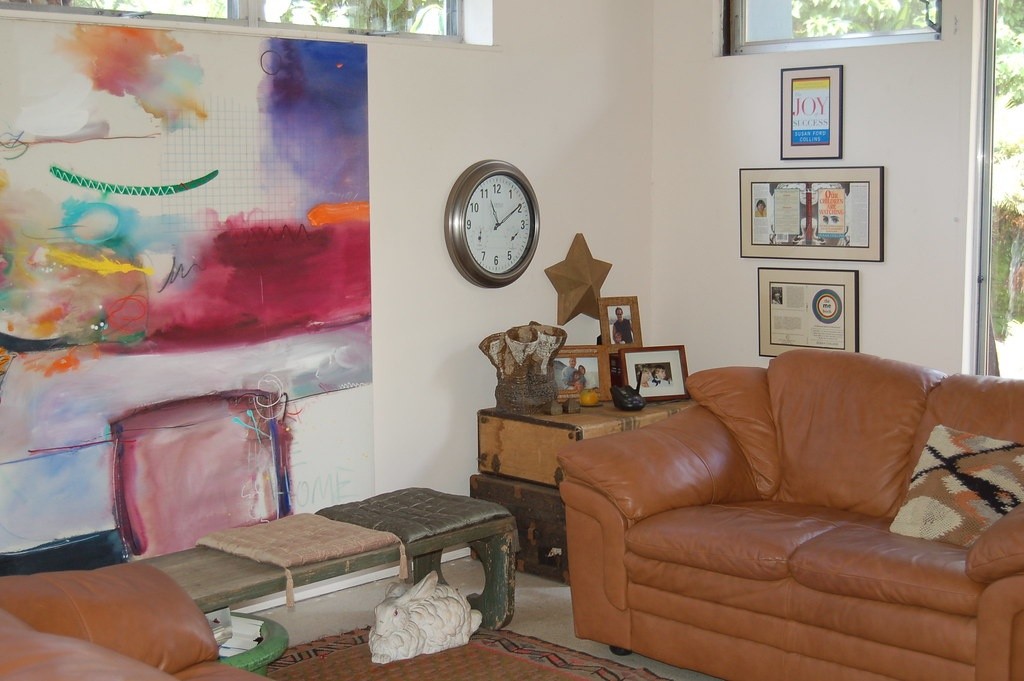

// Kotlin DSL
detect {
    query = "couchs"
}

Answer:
[556,347,1024,681]
[1,561,272,681]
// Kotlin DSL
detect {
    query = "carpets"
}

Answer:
[266,622,674,681]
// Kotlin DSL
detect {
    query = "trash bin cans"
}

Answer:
[216,611,290,676]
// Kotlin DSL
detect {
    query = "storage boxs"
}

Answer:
[478,398,697,490]
[470,474,571,588]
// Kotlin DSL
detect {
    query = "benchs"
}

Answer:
[141,487,520,629]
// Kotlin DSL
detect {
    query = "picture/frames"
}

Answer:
[598,296,644,354]
[739,166,885,262]
[618,345,692,402]
[781,65,843,161]
[553,345,614,403]
[758,267,859,358]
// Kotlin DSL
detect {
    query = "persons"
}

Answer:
[772,288,782,304]
[613,307,633,343]
[578,365,587,387]
[635,363,673,387]
[573,371,584,390]
[615,333,625,344]
[562,358,577,390]
[754,200,767,217]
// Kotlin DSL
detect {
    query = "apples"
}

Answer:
[580,390,600,404]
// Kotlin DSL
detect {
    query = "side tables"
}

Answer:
[218,612,289,676]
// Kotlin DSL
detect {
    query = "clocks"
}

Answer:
[444,158,541,288]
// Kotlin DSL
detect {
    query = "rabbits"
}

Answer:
[368,570,482,665]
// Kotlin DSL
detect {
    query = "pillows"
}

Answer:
[888,424,1024,547]
